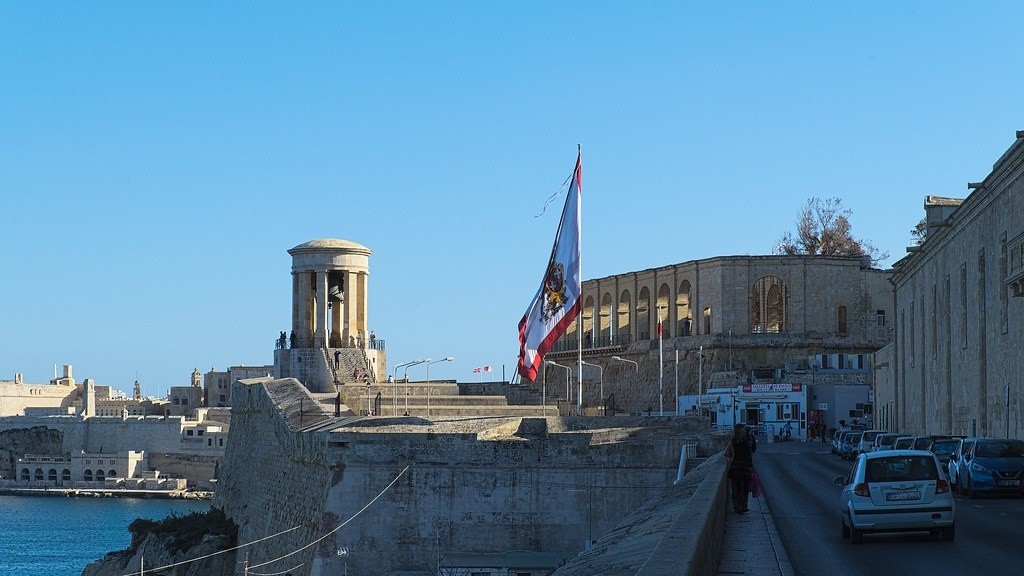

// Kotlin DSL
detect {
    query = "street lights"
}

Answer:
[577,360,603,416]
[404,358,431,416]
[426,357,453,418]
[367,382,371,414]
[394,358,419,416]
[612,356,639,417]
[545,361,573,402]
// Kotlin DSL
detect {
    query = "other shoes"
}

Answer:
[738,508,749,515]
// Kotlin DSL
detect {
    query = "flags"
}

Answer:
[517,153,582,382]
[473,365,491,374]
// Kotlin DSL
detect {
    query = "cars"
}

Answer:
[835,450,957,544]
[831,429,1024,495]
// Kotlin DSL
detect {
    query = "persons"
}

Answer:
[353,368,369,384]
[366,411,410,416]
[358,332,363,348]
[725,423,756,514]
[388,375,393,383]
[370,331,376,348]
[778,420,793,442]
[279,330,296,349]
[334,349,341,369]
[807,421,828,445]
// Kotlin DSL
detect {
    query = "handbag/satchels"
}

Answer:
[747,471,762,498]
